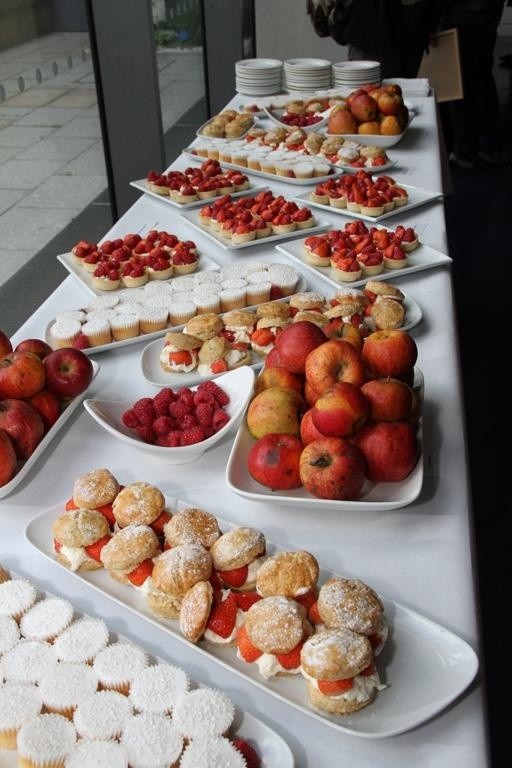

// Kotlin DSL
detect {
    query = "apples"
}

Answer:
[247,319,419,500]
[0,330,93,487]
[329,83,409,135]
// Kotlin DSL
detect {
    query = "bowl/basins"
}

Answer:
[82,362,256,466]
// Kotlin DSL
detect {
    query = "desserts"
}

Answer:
[70,229,200,289]
[194,128,391,178]
[311,170,408,218]
[0,468,389,768]
[204,101,264,136]
[144,159,248,204]
[303,219,419,282]
[278,97,332,128]
[198,191,315,244]
[50,261,406,377]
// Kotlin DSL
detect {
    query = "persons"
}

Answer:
[344,1,438,83]
[439,1,508,168]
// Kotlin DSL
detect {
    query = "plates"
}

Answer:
[231,59,283,96]
[138,289,424,388]
[178,201,329,251]
[181,147,343,187]
[226,361,426,513]
[322,110,419,149]
[283,57,333,91]
[237,102,273,119]
[0,360,102,501]
[262,107,337,130]
[193,117,257,142]
[382,76,431,95]
[274,225,453,290]
[128,178,270,209]
[293,178,444,223]
[27,486,481,739]
[1,570,296,765]
[331,60,382,87]
[333,147,398,173]
[41,261,309,355]
[61,231,222,298]
